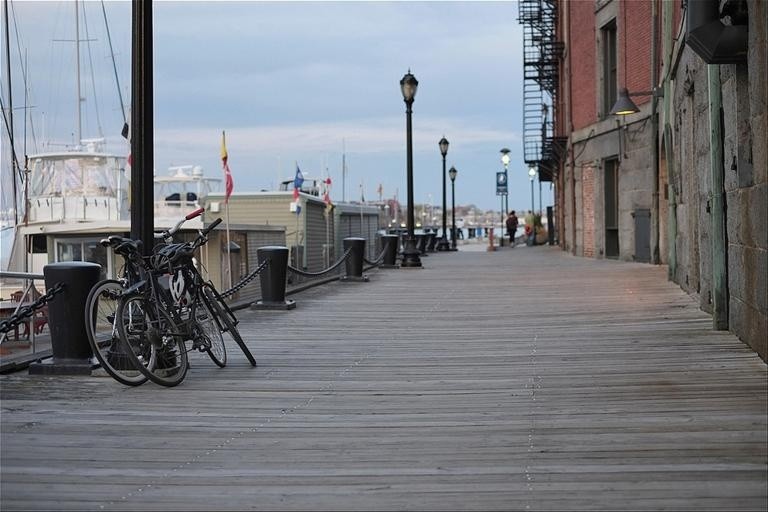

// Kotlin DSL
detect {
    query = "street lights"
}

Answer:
[423,132,460,252]
[500,146,511,215]
[399,67,423,268]
[528,161,537,215]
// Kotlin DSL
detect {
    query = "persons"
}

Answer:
[455,218,464,240]
[506,210,518,248]
[524,210,535,247]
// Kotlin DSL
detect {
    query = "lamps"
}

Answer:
[609,88,663,115]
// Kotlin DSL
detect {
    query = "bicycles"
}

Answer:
[82,206,228,385]
[112,216,258,387]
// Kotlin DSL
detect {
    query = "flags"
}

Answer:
[120,107,131,182]
[293,166,305,216]
[322,177,332,204]
[221,130,233,205]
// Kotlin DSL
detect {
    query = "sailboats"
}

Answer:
[1,2,223,341]
